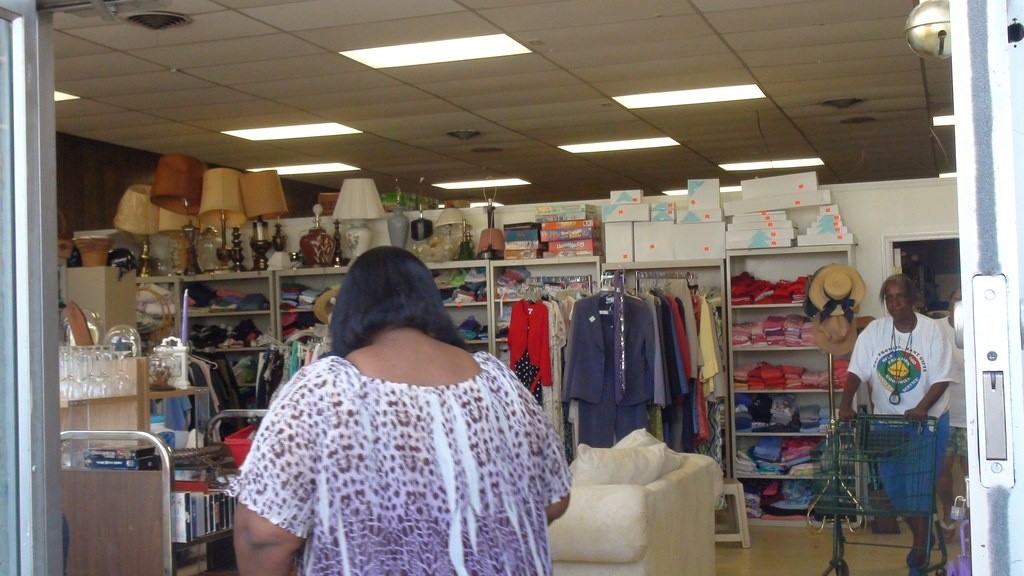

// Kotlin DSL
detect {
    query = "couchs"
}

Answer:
[549,453,724,576]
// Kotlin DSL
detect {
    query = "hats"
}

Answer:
[313,288,339,325]
[813,311,858,357]
[808,263,867,324]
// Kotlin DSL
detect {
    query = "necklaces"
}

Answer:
[889,323,913,404]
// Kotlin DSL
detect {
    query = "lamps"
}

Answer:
[113,153,503,275]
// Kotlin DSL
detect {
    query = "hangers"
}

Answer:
[519,270,701,303]
[186,333,332,370]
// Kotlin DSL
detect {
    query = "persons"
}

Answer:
[225,246,573,576]
[838,274,971,566]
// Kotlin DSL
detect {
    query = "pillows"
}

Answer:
[570,428,686,484]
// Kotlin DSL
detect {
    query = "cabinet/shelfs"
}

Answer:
[136,271,278,405]
[725,245,860,527]
[61,356,268,576]
[276,260,492,385]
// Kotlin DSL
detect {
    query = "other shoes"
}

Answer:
[905,532,936,576]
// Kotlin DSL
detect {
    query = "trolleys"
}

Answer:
[810,413,947,576]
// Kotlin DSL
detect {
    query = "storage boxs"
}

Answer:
[64,265,136,341]
[503,172,858,262]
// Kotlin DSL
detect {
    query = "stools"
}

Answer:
[715,478,751,548]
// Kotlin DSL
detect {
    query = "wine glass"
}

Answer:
[59,345,134,400]
